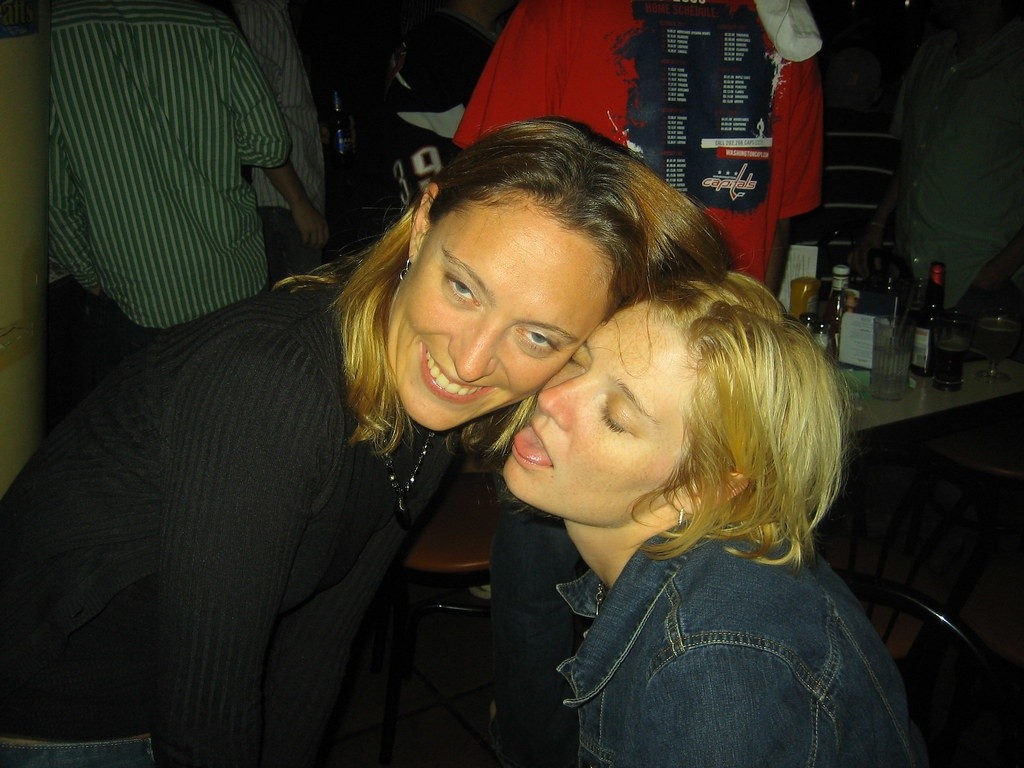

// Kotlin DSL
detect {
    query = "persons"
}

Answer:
[43,0,1024,538]
[0,118,732,768]
[503,270,931,768]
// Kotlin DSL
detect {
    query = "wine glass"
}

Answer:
[975,302,1022,382]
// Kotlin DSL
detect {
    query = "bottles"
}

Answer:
[829,264,850,360]
[910,262,946,377]
[867,242,899,293]
[906,274,927,317]
[800,311,818,336]
[329,89,352,161]
[813,321,830,351]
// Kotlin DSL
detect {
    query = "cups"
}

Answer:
[932,311,975,390]
[869,315,916,399]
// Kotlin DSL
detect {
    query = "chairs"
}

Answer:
[819,439,993,768]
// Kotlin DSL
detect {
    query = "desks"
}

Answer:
[473,319,1024,768]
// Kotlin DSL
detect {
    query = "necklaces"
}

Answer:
[595,580,606,619]
[383,430,437,511]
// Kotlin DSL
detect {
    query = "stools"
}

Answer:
[362,471,500,766]
